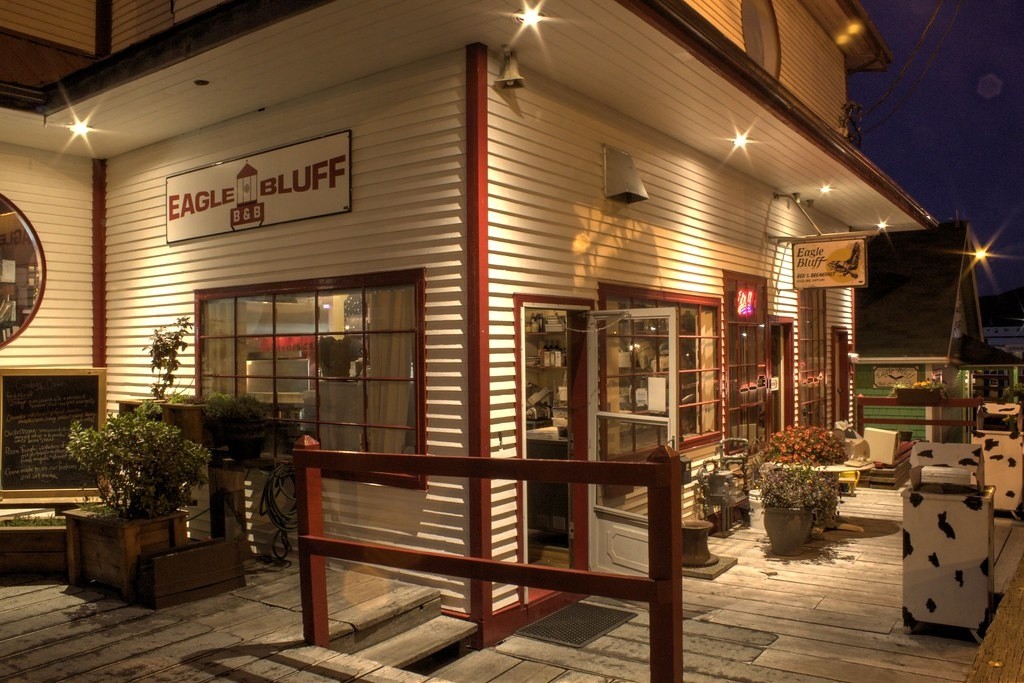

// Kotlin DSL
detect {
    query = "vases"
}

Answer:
[680,519,719,567]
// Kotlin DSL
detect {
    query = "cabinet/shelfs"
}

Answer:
[525,331,567,370]
[899,485,998,645]
[970,430,1024,520]
[526,426,569,538]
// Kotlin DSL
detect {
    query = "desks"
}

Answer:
[777,457,875,537]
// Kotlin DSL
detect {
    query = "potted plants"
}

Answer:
[114,317,193,422]
[159,393,229,450]
[760,465,839,556]
[205,391,268,463]
[888,381,944,405]
[61,412,213,601]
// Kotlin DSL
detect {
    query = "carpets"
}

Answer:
[515,601,639,650]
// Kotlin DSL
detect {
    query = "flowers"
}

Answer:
[764,425,848,466]
